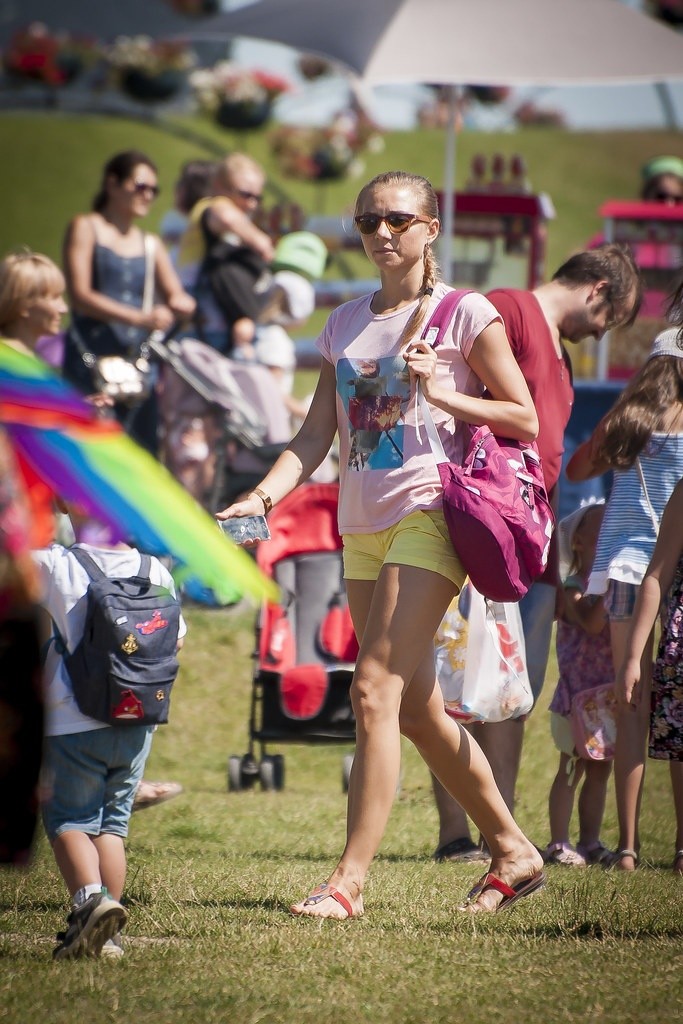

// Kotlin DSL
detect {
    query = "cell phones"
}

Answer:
[218,516,271,544]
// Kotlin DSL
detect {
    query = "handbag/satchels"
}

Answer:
[570,683,618,763]
[80,349,153,405]
[413,289,555,604]
[462,581,534,723]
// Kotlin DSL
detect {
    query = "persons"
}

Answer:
[432,248,645,864]
[29,492,187,960]
[580,155,683,382]
[214,171,543,919]
[546,679,556,680]
[566,327,683,874]
[621,279,683,875]
[65,151,198,436]
[0,254,182,875]
[556,497,617,866]
[162,154,329,518]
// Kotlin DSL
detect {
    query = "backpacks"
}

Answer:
[53,547,180,725]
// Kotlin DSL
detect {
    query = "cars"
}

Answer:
[557,379,631,523]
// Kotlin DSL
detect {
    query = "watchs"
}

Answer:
[248,489,273,515]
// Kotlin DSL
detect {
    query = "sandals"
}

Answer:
[546,841,587,868]
[577,840,618,868]
[616,849,639,874]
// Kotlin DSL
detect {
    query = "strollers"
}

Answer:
[227,453,406,794]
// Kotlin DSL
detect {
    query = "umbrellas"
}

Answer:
[167,0,683,285]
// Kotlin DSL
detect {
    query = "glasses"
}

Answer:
[354,215,434,235]
[240,191,264,200]
[655,190,683,203]
[134,181,159,195]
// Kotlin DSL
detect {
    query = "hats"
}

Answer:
[274,231,328,279]
[557,503,604,584]
[650,327,683,359]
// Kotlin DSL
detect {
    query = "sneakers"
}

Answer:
[51,886,127,959]
[102,939,124,963]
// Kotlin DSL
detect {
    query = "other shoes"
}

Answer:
[130,781,186,815]
[433,837,494,868]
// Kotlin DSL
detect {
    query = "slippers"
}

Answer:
[456,869,546,918]
[289,883,354,919]
